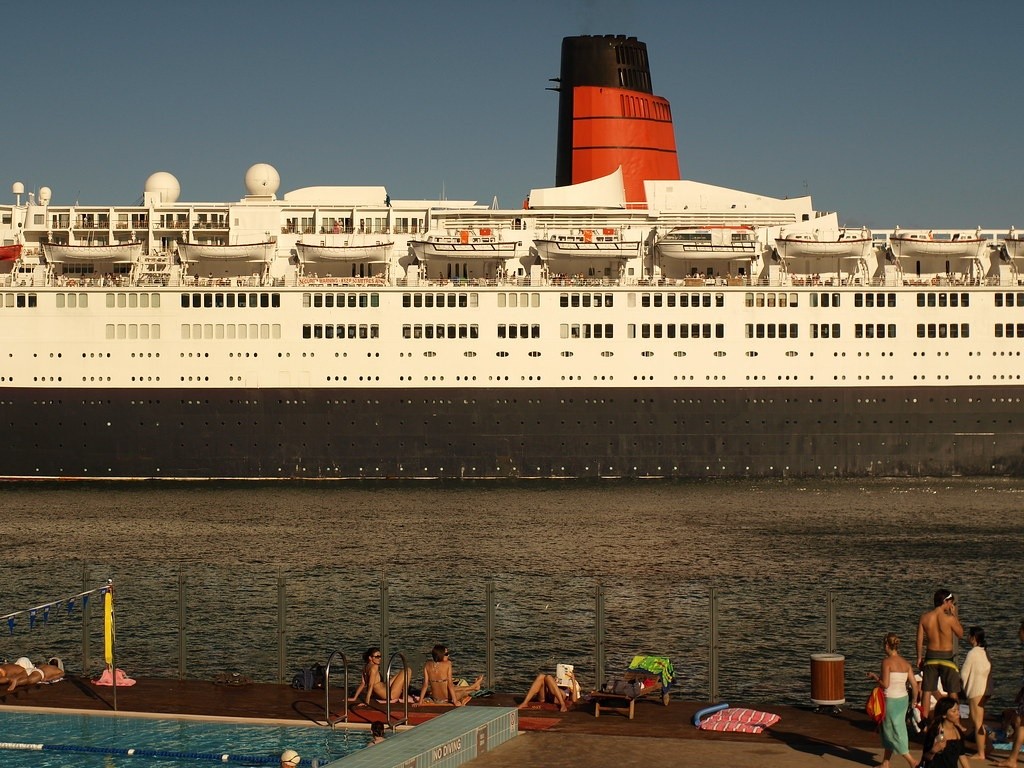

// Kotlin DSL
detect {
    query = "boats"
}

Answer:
[531,234,641,259]
[294,242,395,265]
[774,227,872,258]
[407,236,518,259]
[176,240,277,264]
[40,241,143,265]
[886,233,989,260]
[0,245,24,261]
[654,225,766,259]
[1005,238,1024,256]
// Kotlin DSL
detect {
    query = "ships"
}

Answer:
[0,35,1024,484]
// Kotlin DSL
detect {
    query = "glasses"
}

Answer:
[444,652,449,656]
[372,655,382,659]
[943,592,953,601]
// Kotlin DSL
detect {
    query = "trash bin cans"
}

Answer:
[809,653,845,705]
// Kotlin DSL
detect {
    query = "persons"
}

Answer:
[0,659,65,691]
[194,274,199,287]
[342,647,412,706]
[54,271,123,287]
[367,720,386,747]
[907,671,1017,768]
[386,194,392,207]
[959,626,994,760]
[517,673,577,712]
[865,632,921,768]
[208,272,213,287]
[219,271,229,287]
[281,749,300,768]
[411,644,483,706]
[306,270,998,287]
[986,617,1024,768]
[917,589,968,733]
[53,215,427,234]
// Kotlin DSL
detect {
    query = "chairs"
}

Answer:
[594,654,675,720]
[902,279,930,288]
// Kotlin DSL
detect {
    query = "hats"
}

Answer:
[281,750,300,766]
[370,721,384,730]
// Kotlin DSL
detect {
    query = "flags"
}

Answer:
[602,229,613,235]
[479,229,490,235]
[460,231,469,244]
[583,230,593,244]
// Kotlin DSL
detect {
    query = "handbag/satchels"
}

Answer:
[304,663,326,689]
[599,678,641,698]
[292,673,305,689]
[865,679,886,724]
